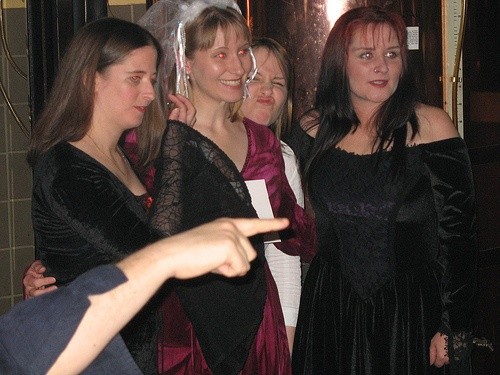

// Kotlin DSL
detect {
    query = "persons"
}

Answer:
[284,5,480,375]
[0,1,316,375]
